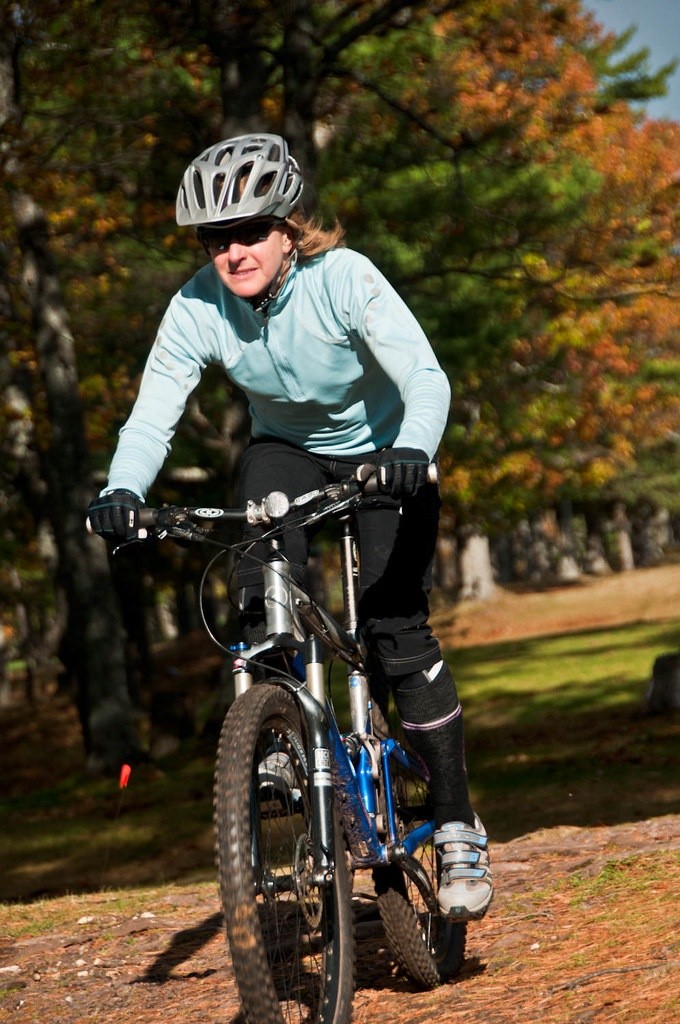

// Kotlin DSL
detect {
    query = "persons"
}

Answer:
[88,132,495,919]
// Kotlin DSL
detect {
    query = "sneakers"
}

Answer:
[433,811,494,918]
[257,752,296,801]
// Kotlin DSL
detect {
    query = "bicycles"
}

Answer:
[81,464,470,1024]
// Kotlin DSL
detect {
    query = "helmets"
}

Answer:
[176,132,305,227]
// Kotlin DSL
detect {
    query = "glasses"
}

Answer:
[194,218,285,251]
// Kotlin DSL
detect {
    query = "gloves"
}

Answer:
[88,489,146,542]
[356,447,430,500]
